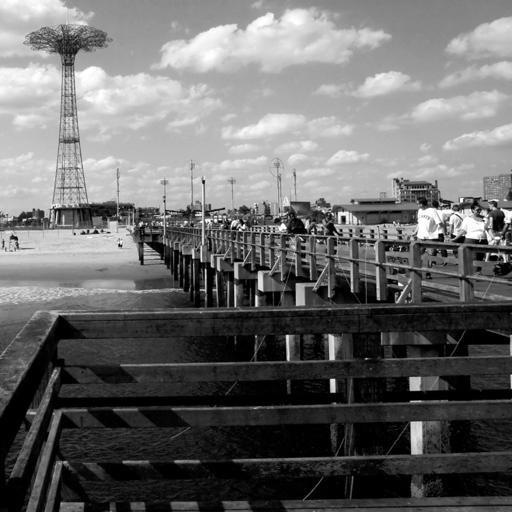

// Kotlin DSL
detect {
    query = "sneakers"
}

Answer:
[431,261,458,266]
[425,272,434,280]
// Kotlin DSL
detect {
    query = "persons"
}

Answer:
[120,239,123,248]
[117,238,120,248]
[138,212,343,263]
[409,196,511,280]
[2,239,6,250]
